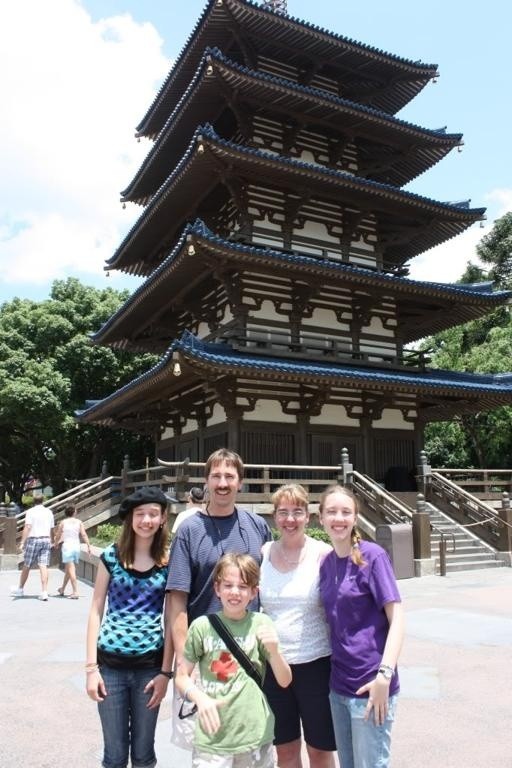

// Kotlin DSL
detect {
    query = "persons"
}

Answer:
[254,469,335,768]
[84,486,177,768]
[170,449,272,768]
[313,484,408,768]
[171,487,204,534]
[51,505,93,600]
[173,553,294,766]
[14,487,57,602]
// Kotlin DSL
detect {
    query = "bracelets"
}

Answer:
[182,680,197,705]
[157,669,174,679]
[84,662,101,673]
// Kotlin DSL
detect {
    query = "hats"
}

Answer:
[118,487,167,520]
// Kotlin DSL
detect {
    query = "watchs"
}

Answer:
[377,664,395,679]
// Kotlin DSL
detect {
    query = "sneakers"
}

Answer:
[10,588,79,600]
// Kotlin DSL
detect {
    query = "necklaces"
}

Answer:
[277,537,309,563]
